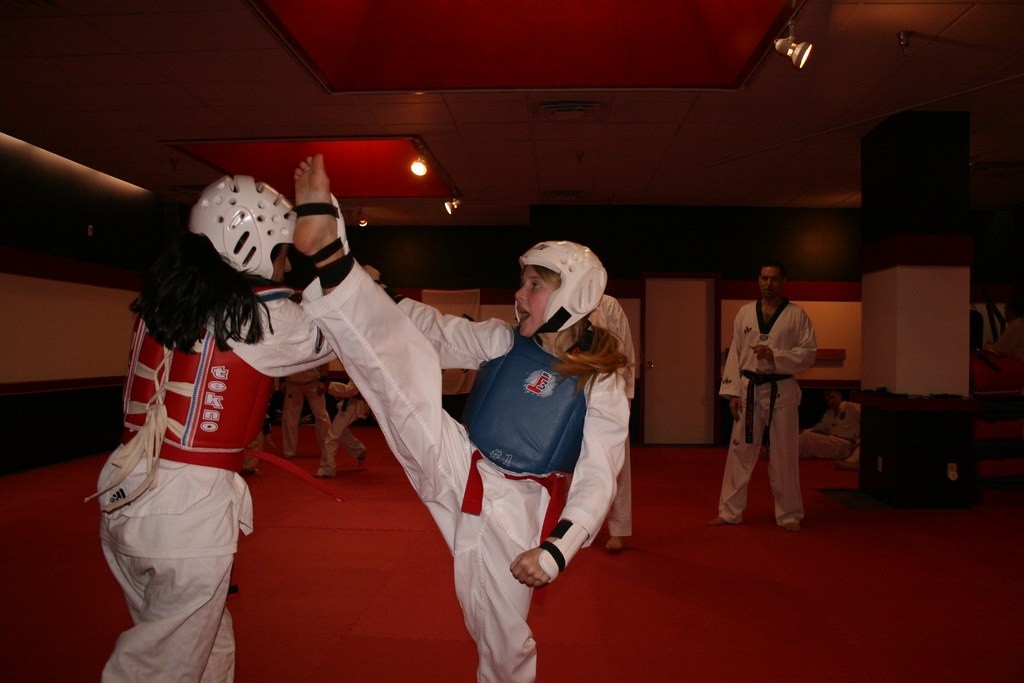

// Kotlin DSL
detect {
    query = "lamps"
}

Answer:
[773,19,812,67]
[410,155,428,175]
[445,198,462,213]
[357,211,367,226]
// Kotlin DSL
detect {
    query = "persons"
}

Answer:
[707,262,817,532]
[573,294,637,554]
[239,366,370,478]
[799,389,861,471]
[293,154,636,683]
[95,175,388,683]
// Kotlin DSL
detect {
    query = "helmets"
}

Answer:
[514,240,608,334]
[188,175,298,280]
